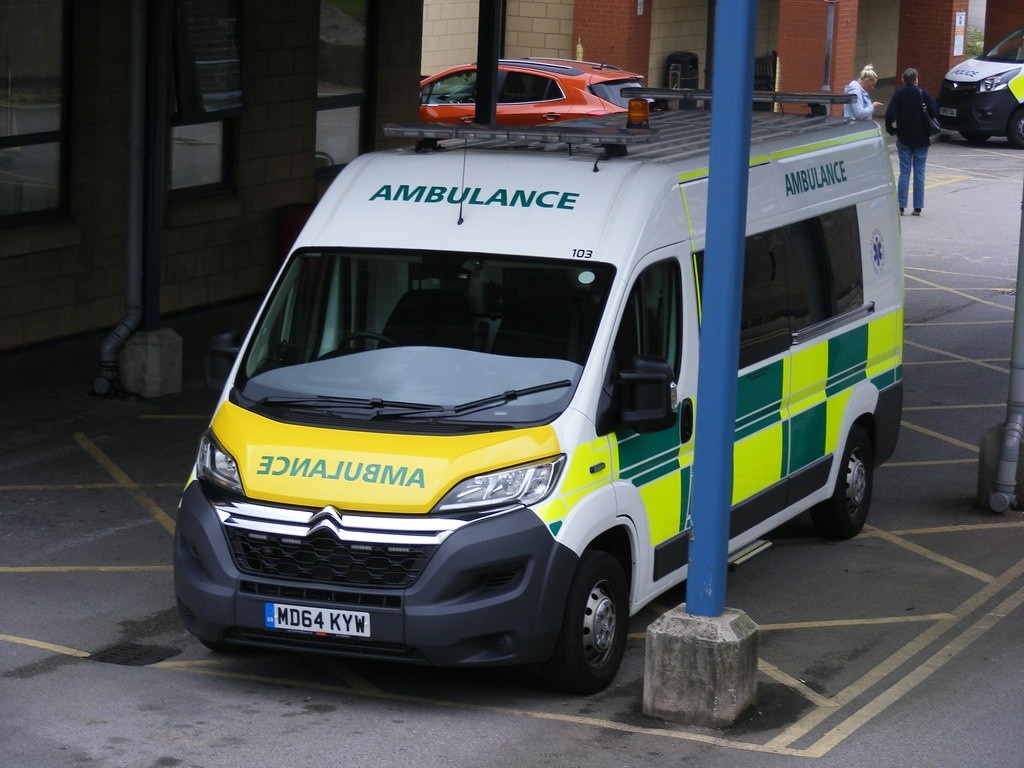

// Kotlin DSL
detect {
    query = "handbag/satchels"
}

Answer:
[922,102,942,137]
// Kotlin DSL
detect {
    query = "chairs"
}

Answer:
[531,77,552,101]
[498,77,525,103]
[375,286,475,352]
[491,283,602,365]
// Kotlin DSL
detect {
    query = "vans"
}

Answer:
[935,26,1024,150]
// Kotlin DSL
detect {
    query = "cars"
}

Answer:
[419,57,654,126]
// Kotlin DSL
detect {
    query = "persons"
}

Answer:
[885,68,940,216]
[844,64,880,121]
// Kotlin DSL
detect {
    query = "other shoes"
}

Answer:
[912,207,922,216]
[899,207,905,216]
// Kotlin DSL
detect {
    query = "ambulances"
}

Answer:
[175,104,904,701]
